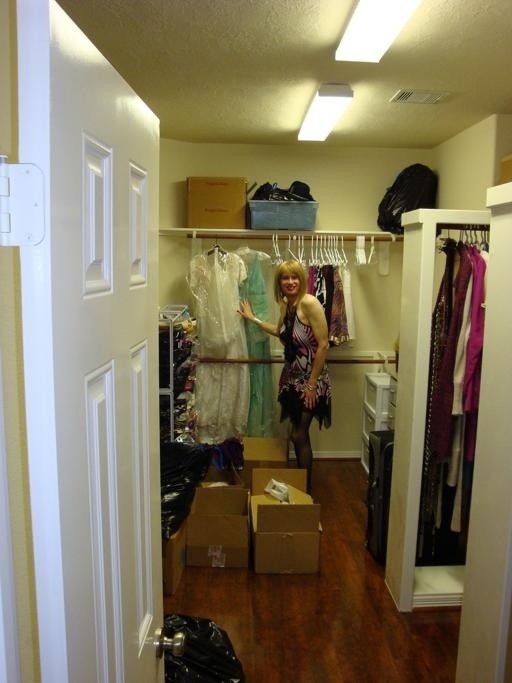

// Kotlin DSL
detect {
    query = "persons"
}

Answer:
[237,259,332,495]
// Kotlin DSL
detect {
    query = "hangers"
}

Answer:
[445,224,488,250]
[206,233,250,256]
[270,233,378,270]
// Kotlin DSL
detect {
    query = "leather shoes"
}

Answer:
[159,314,201,447]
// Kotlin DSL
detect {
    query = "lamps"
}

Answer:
[296,0,420,142]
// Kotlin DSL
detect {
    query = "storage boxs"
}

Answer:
[185,174,319,231]
[162,434,322,597]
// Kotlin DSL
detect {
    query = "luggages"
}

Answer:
[367,429,460,567]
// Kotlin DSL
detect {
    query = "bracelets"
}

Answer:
[254,316,265,326]
[303,383,318,392]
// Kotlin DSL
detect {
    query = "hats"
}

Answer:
[279,181,314,201]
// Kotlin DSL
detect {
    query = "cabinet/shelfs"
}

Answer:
[361,372,397,477]
[158,303,191,442]
